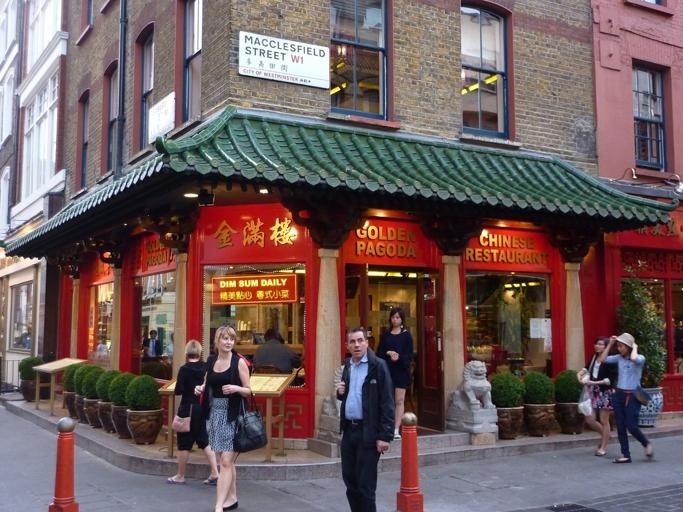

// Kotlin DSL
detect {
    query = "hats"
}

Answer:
[616,332,635,348]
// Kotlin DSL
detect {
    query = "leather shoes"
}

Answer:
[613,457,632,463]
[223,501,238,511]
[594,442,607,456]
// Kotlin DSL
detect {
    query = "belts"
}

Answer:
[346,418,361,425]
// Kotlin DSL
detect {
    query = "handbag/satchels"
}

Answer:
[632,385,650,405]
[171,415,191,432]
[233,392,268,452]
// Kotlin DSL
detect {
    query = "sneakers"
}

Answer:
[395,434,401,439]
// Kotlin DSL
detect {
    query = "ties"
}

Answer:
[152,340,154,355]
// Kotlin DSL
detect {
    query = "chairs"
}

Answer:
[253,364,283,373]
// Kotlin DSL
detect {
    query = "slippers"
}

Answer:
[206,478,217,485]
[167,477,184,484]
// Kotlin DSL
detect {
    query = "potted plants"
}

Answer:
[488,260,666,437]
[16,357,164,445]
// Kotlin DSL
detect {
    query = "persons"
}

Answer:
[142,330,163,360]
[374,307,413,439]
[166,340,219,485]
[96,335,109,359]
[336,328,396,512]
[674,320,683,374]
[165,332,173,354]
[577,335,619,456]
[596,332,654,463]
[18,324,32,349]
[251,328,301,374]
[194,325,252,512]
